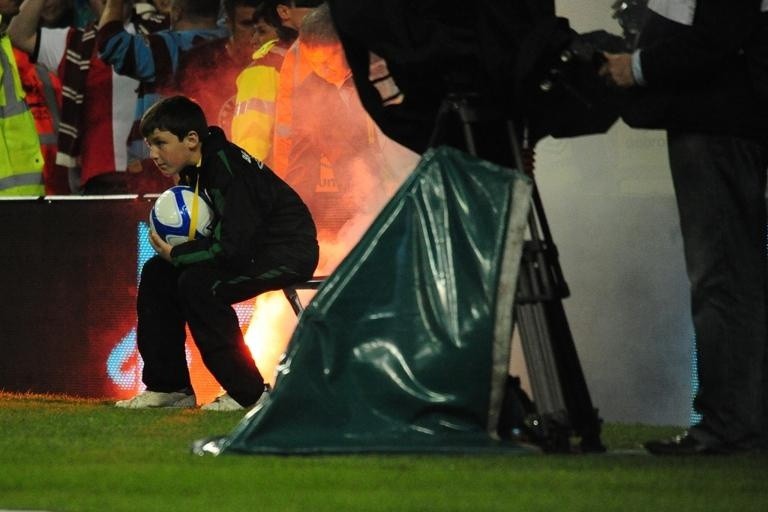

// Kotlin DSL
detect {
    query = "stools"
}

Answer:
[282,275,331,319]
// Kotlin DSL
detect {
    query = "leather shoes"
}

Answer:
[642,431,737,455]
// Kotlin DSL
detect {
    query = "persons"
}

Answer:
[114,96,319,412]
[1,0,413,230]
[599,0,768,456]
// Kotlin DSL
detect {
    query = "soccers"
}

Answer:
[149,186,213,247]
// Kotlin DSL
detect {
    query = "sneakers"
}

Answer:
[113,388,197,411]
[200,385,272,414]
